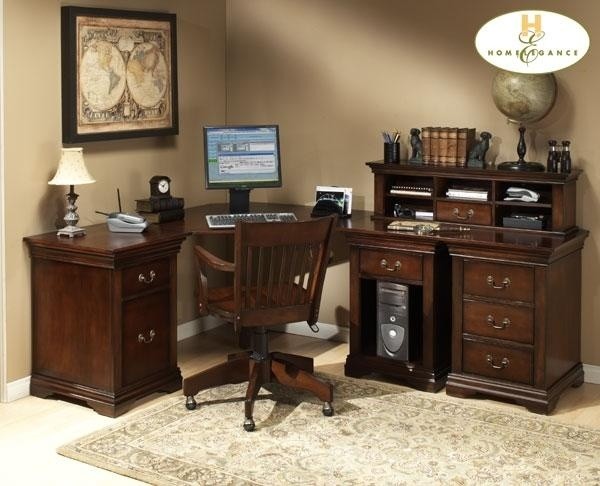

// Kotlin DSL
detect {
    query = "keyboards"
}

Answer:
[205,211,299,230]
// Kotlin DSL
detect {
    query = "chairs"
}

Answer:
[182,214,341,432]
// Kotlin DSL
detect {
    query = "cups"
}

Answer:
[384,142,400,164]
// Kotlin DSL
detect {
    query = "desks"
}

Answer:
[182,202,370,350]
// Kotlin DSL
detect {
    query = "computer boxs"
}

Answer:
[375,279,410,363]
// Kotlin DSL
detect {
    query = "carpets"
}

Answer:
[55,369,600,486]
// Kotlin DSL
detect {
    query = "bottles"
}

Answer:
[547,140,571,173]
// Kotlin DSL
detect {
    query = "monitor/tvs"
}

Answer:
[202,124,282,213]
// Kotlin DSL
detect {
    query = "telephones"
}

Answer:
[97,211,150,233]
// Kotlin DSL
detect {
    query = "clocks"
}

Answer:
[149,175,172,200]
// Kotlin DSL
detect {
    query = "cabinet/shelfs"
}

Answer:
[343,215,450,393]
[445,229,590,415]
[23,222,192,418]
[364,159,583,236]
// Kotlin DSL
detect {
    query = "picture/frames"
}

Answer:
[61,6,179,143]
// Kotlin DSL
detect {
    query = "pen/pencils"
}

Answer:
[382,131,402,143]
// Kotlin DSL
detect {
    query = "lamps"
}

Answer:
[48,147,97,240]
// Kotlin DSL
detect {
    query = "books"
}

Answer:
[419,125,477,168]
[389,182,434,196]
[442,183,490,201]
